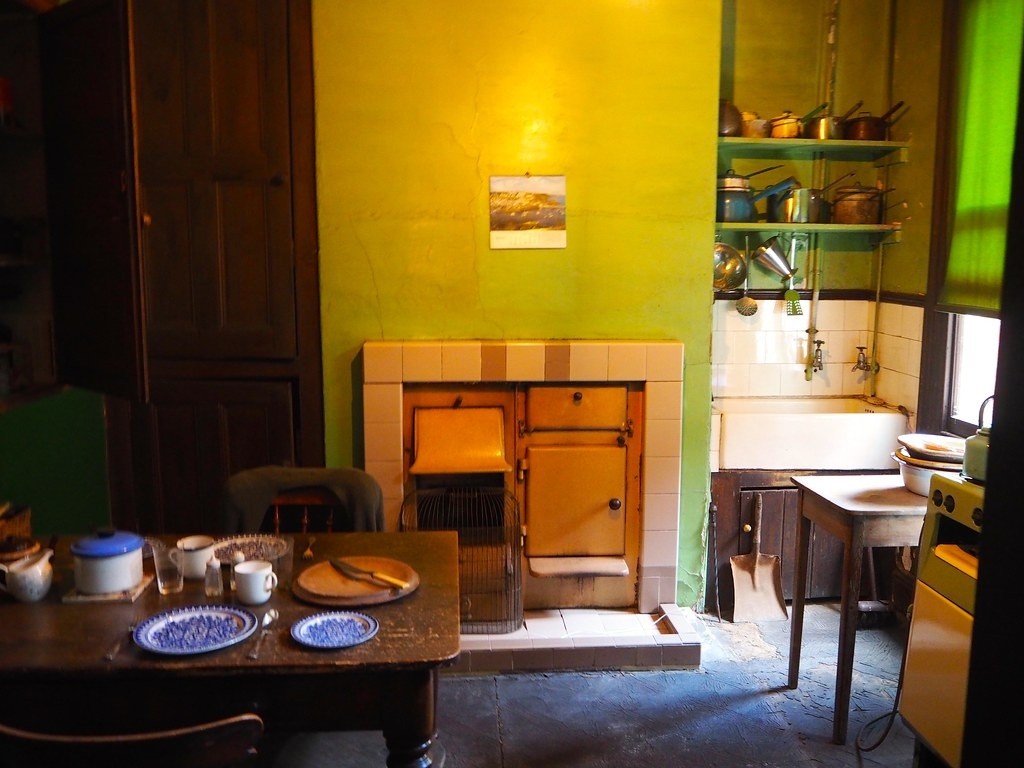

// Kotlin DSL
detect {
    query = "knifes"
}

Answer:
[327,554,409,590]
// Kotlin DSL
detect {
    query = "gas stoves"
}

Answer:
[916,472,986,615]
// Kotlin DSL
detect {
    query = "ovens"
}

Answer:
[898,580,979,768]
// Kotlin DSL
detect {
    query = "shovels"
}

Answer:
[729,493,791,623]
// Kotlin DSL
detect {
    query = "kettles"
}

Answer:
[963,394,994,481]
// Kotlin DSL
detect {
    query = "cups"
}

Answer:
[151,543,184,594]
[234,560,278,605]
[751,236,799,283]
[168,535,214,578]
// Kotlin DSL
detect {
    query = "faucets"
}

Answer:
[812,339,826,370]
[851,346,880,374]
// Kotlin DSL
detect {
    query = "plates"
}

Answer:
[292,556,420,605]
[133,604,258,654]
[291,611,380,648]
[142,537,163,558]
[212,534,291,563]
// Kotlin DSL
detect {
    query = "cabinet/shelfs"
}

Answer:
[0,0,326,538]
[710,469,891,599]
[718,134,908,242]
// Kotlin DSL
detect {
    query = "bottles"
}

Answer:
[203,551,246,597]
[719,99,758,136]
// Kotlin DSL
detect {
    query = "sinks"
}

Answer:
[711,396,909,472]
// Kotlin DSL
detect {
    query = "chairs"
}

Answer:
[0,711,265,768]
[226,468,384,534]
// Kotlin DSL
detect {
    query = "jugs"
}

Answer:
[0,548,54,601]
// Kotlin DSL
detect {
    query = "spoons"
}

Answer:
[248,608,279,658]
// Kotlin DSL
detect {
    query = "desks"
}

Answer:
[0,532,462,768]
[790,474,928,744]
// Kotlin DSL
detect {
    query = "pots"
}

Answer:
[832,183,896,224]
[767,171,856,223]
[716,169,796,223]
[740,101,904,141]
[69,530,144,594]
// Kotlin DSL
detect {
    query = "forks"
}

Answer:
[303,537,316,559]
[105,615,145,661]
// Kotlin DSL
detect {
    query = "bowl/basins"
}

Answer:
[890,452,962,497]
